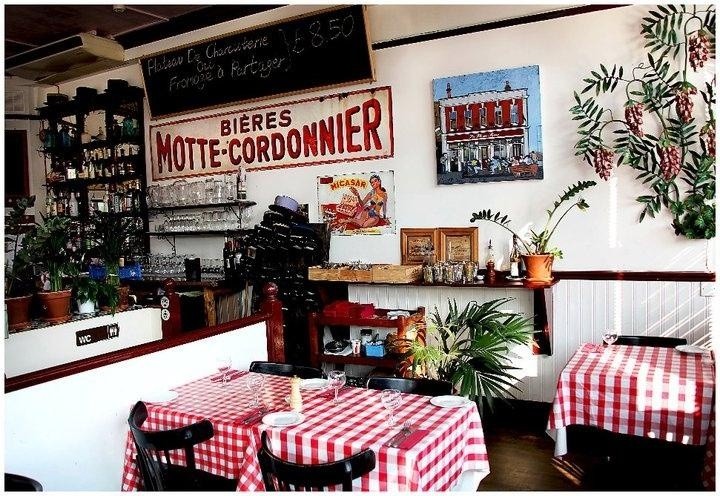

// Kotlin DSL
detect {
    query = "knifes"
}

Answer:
[393,426,420,448]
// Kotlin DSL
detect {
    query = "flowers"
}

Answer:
[395,296,541,421]
[569,5,716,239]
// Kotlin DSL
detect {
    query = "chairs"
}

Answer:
[364,377,454,394]
[257,431,376,491]
[128,402,237,490]
[5,472,43,491]
[603,335,687,347]
[248,361,323,379]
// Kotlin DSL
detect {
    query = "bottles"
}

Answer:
[290,375,303,409]
[163,214,202,231]
[45,113,143,253]
[148,166,246,206]
[510,234,522,278]
[223,235,244,274]
[485,239,496,279]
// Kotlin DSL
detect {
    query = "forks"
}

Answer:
[386,420,412,448]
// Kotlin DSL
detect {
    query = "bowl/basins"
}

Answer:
[325,340,349,352]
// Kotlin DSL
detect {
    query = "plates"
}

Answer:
[675,345,704,354]
[142,390,178,405]
[506,276,523,281]
[429,396,468,409]
[300,378,330,390]
[262,412,305,427]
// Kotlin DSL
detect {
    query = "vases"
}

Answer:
[453,388,458,395]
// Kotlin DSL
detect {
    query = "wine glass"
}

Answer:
[328,370,346,404]
[217,356,233,388]
[602,328,618,355]
[247,373,264,409]
[381,389,403,426]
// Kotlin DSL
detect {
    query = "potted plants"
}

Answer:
[5,196,127,328]
[467,178,597,284]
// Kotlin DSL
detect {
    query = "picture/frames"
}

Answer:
[399,225,479,265]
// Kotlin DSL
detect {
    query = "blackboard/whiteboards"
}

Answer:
[137,4,378,122]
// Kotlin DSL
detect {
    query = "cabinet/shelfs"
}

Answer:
[306,302,425,385]
[144,200,256,256]
[37,86,151,276]
[304,277,562,375]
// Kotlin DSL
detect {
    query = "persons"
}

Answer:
[339,174,388,227]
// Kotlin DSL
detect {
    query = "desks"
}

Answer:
[46,269,256,331]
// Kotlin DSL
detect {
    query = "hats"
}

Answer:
[72,87,97,99]
[269,196,299,217]
[104,80,128,93]
[44,93,68,104]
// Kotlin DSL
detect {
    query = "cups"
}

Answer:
[352,339,363,355]
[202,211,240,229]
